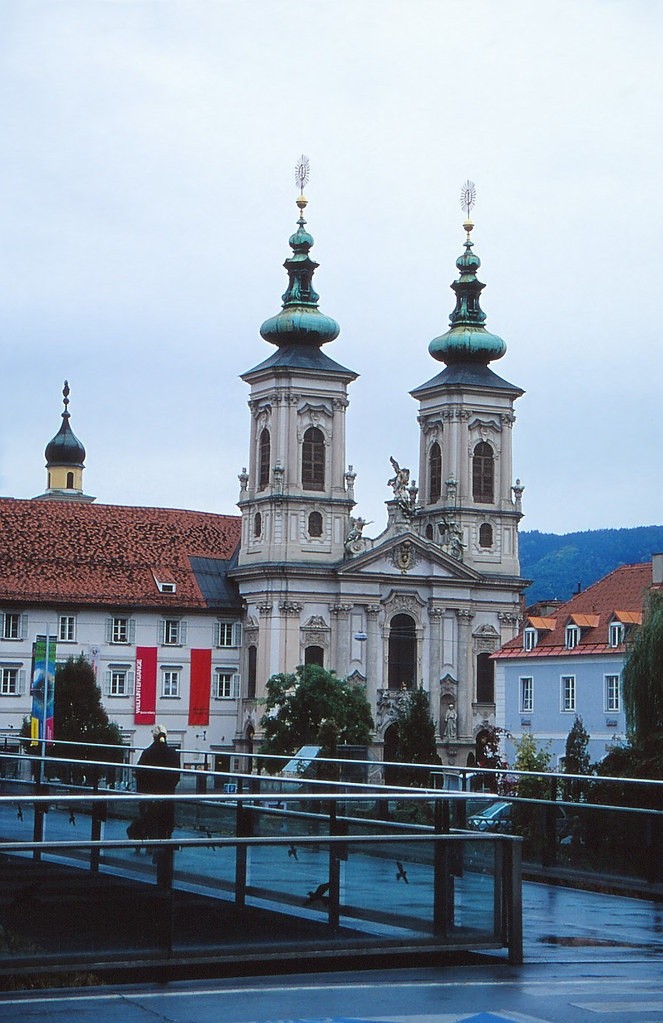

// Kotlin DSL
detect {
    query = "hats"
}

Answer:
[151,724,168,736]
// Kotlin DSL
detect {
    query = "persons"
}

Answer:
[442,703,458,740]
[137,726,181,854]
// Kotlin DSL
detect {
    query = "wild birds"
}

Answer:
[302,882,332,907]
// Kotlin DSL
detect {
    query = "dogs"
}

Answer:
[126,817,153,855]
